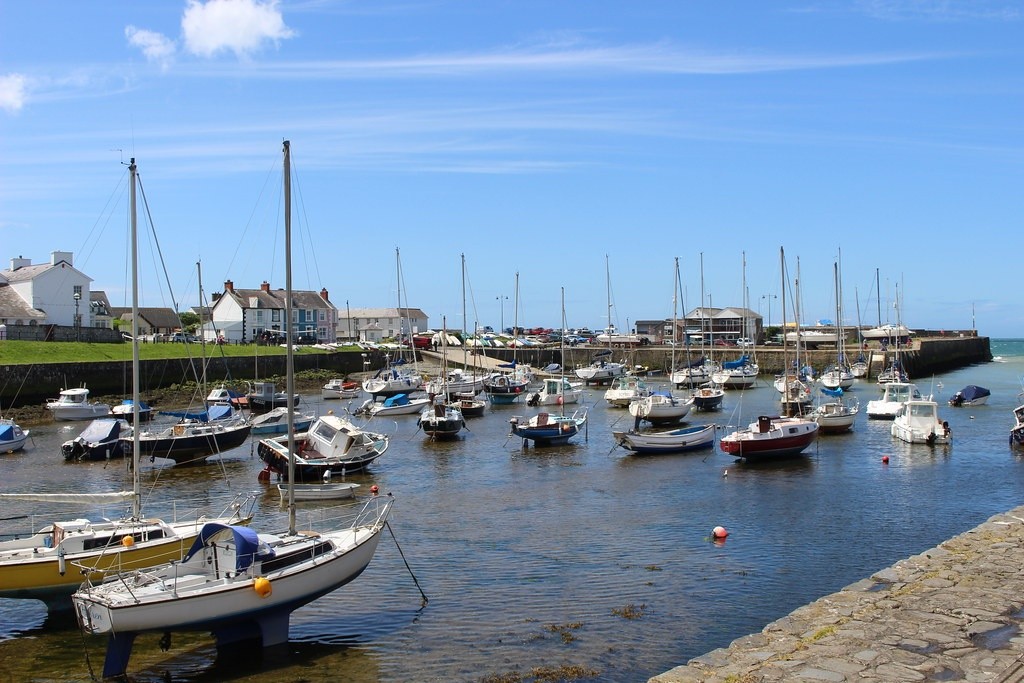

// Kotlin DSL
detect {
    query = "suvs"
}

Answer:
[736,338,753,347]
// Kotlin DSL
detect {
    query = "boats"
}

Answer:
[60,405,136,461]
[612,416,717,456]
[45,371,111,422]
[205,369,300,412]
[261,404,390,481]
[319,378,362,399]
[0,416,29,454]
[111,398,152,422]
[866,373,1024,456]
[278,482,362,503]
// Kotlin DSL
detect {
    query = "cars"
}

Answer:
[297,336,323,344]
[714,337,736,346]
[506,326,593,343]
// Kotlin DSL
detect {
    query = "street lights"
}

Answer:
[73,293,80,327]
[762,293,777,340]
[496,294,508,334]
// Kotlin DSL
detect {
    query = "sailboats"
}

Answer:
[576,246,916,456]
[509,287,588,447]
[0,128,395,679]
[354,243,532,437]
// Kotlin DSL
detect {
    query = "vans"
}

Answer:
[168,331,195,341]
[402,336,433,350]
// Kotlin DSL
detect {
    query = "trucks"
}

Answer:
[477,326,493,333]
[689,335,708,346]
